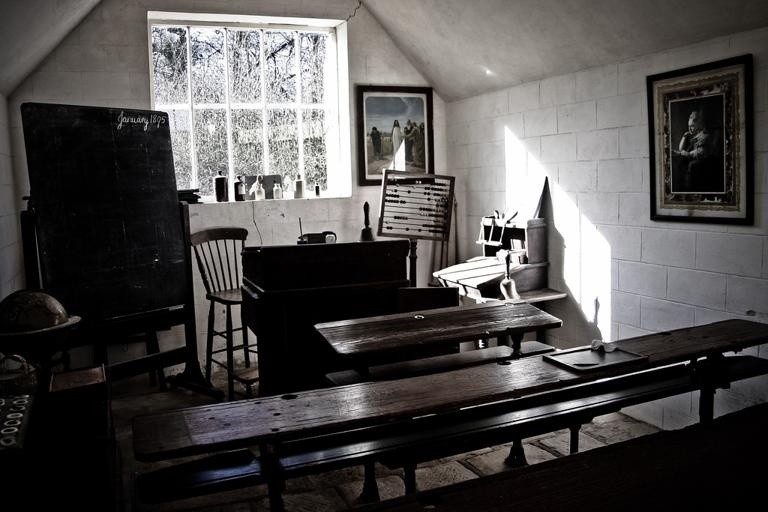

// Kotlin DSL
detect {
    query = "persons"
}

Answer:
[672,110,723,191]
[368,119,425,167]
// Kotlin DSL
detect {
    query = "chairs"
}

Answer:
[188,225,259,401]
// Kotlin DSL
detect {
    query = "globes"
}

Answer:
[0,290,82,394]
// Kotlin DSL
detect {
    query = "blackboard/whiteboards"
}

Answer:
[21,102,192,325]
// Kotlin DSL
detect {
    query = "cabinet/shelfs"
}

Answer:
[0,362,127,511]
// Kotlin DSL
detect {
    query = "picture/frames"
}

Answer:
[644,51,755,227]
[353,83,434,186]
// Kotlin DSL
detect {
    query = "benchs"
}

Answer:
[137,354,764,508]
[326,340,556,386]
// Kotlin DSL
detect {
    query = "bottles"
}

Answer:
[213,168,323,202]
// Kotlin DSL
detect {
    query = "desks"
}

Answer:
[366,401,764,510]
[183,319,764,509]
[315,300,564,381]
